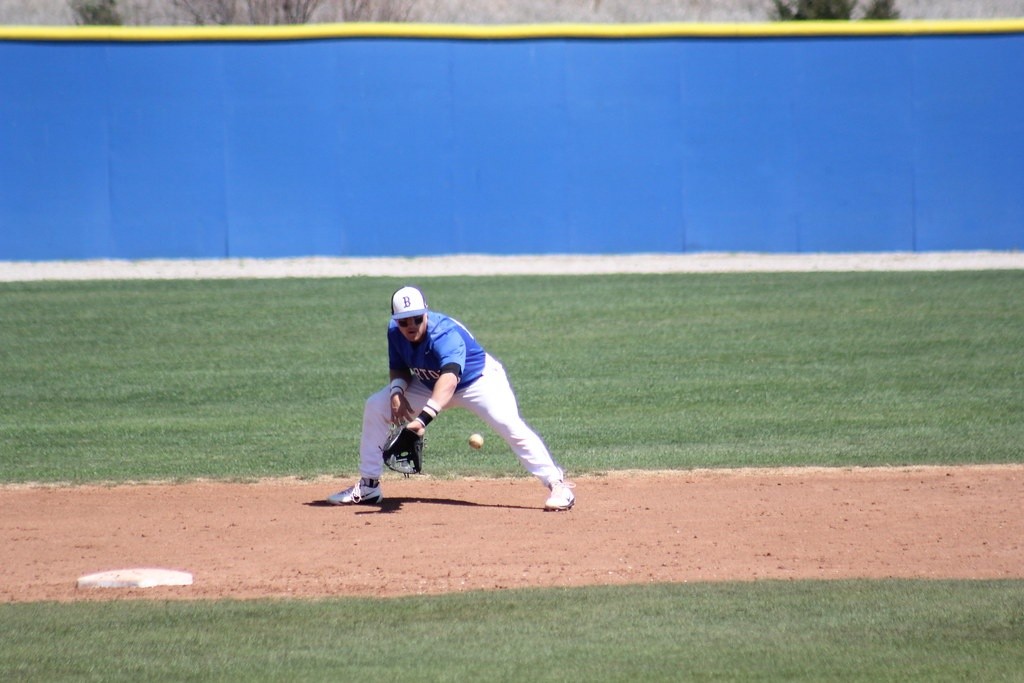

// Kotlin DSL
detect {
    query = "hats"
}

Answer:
[387,286,427,319]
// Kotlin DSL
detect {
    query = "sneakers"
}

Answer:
[326,483,382,505]
[546,483,574,511]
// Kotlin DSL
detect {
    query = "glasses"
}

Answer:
[397,317,423,328]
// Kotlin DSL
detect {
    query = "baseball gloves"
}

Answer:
[383,426,424,475]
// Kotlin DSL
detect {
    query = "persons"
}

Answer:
[327,287,576,512]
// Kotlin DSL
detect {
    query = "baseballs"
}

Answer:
[469,434,484,450]
